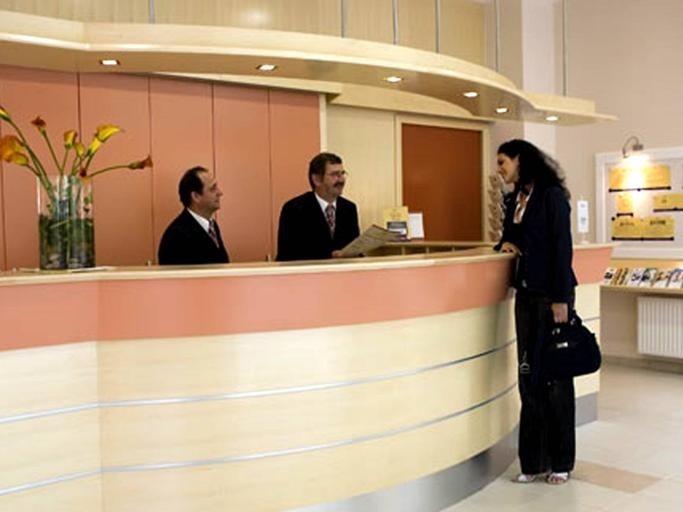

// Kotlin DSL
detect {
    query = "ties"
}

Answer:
[326,204,336,236]
[209,221,219,248]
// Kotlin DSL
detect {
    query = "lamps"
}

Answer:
[622,134,645,160]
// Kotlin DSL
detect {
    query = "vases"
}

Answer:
[36,174,96,270]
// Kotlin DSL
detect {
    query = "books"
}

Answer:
[600,266,683,290]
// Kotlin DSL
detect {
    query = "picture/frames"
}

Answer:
[595,146,682,262]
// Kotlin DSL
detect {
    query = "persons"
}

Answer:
[276,152,366,263]
[494,137,576,485]
[158,166,229,266]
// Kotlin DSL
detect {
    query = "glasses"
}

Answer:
[323,170,349,181]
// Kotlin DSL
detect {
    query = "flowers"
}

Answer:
[0,102,153,254]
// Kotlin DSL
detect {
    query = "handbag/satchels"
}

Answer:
[544,309,601,379]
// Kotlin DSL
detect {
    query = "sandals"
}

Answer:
[548,471,570,485]
[511,466,552,483]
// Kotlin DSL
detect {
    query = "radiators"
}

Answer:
[637,298,683,359]
